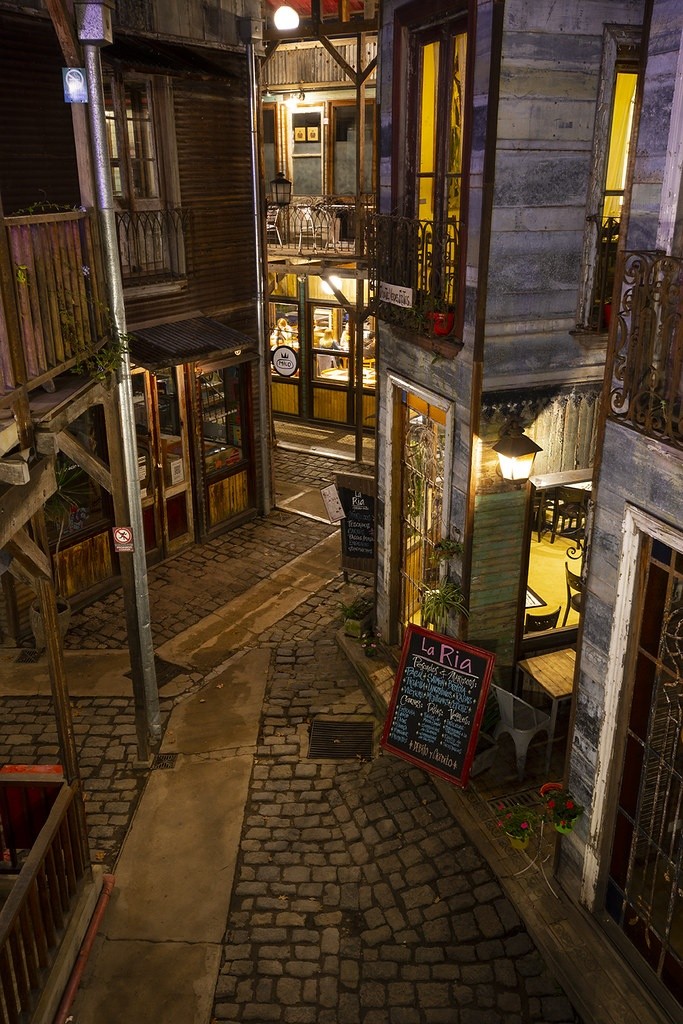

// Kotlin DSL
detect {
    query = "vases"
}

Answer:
[540,783,579,833]
[505,831,529,849]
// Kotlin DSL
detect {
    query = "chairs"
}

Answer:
[490,684,550,769]
[524,489,582,634]
[266,193,375,255]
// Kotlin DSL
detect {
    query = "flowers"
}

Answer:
[496,803,542,838]
[541,791,585,830]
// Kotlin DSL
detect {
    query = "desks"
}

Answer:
[516,648,576,772]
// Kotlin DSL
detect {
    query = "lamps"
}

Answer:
[492,421,543,490]
[265,170,291,205]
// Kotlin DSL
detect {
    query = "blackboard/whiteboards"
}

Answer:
[335,474,378,578]
[380,622,495,787]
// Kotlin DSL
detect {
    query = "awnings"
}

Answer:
[129,317,256,372]
